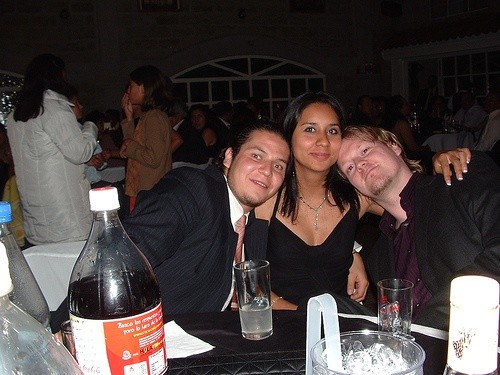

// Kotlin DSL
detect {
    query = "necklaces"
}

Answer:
[297,189,331,230]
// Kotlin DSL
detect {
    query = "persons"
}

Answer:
[264,93,471,315]
[6,54,98,248]
[120,63,172,216]
[0,91,500,253]
[332,123,500,335]
[47,117,290,329]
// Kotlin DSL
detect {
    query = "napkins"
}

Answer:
[162,320,215,359]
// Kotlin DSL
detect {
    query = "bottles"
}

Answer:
[68,187,168,375]
[444,275,499,375]
[256,112,262,120]
[0,242,85,375]
[410,104,418,126]
[0,200,54,362]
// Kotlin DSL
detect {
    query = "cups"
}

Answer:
[60,320,76,360]
[310,330,426,375]
[377,278,414,340]
[234,260,273,340]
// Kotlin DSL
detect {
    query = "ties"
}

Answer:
[228,214,246,311]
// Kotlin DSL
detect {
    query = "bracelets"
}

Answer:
[271,296,281,306]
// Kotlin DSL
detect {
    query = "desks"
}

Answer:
[423,132,475,151]
[48,309,500,375]
